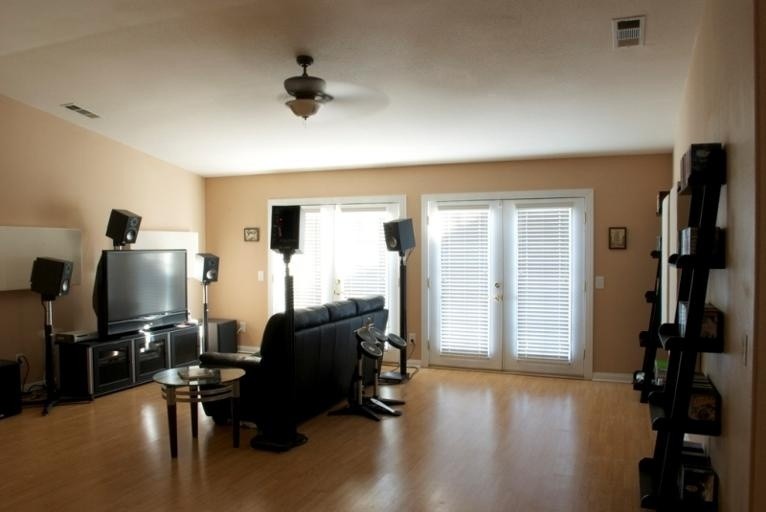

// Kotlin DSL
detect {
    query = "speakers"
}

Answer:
[0,360,20,419]
[192,253,219,283]
[208,318,237,353]
[271,206,304,254]
[30,256,73,296]
[105,209,142,243]
[384,218,416,251]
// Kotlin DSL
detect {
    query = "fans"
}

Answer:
[249,50,391,128]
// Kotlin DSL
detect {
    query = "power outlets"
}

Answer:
[408,333,417,345]
[238,322,246,335]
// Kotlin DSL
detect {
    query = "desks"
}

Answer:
[150,365,239,465]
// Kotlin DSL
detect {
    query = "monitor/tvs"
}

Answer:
[93,249,188,343]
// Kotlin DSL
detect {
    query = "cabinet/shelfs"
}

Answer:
[53,322,201,399]
[639,145,720,512]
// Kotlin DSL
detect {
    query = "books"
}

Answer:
[655,143,726,502]
[177,368,214,380]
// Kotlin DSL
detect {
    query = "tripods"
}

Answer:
[22,297,93,415]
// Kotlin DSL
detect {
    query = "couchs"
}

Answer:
[195,296,390,451]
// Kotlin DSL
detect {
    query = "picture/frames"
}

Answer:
[608,227,627,249]
[244,228,259,242]
